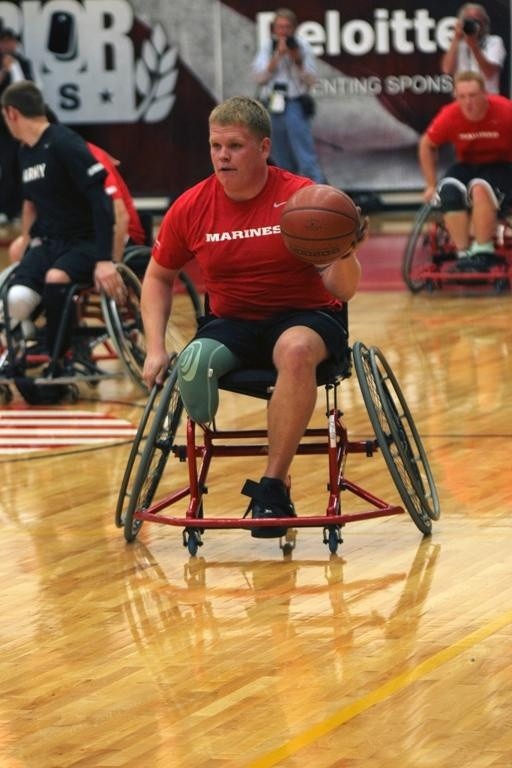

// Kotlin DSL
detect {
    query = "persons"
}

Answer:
[248,8,326,186]
[142,95,361,538]
[417,72,511,273]
[7,138,149,261]
[440,1,507,96]
[0,79,128,390]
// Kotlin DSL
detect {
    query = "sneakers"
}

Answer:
[250,477,297,538]
[449,254,500,286]
[65,360,100,393]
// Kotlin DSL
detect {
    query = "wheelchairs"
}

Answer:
[115,288,442,558]
[0,242,203,406]
[401,193,512,297]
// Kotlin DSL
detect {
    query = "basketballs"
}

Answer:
[279,184,360,263]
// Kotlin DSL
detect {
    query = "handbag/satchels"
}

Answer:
[297,94,317,121]
